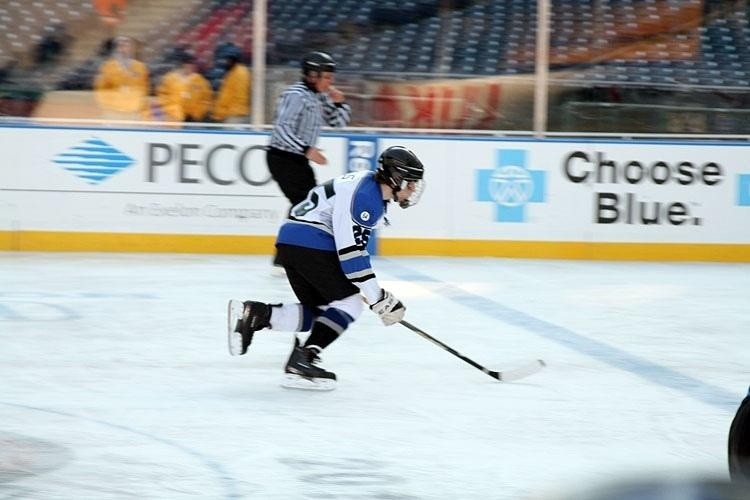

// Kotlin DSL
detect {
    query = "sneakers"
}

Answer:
[286,338,338,379]
[240,300,270,352]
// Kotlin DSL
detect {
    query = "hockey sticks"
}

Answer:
[400,320,547,381]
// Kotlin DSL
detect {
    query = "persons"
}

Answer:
[229,145,425,381]
[264,49,355,267]
[156,48,213,132]
[91,34,154,122]
[210,45,251,132]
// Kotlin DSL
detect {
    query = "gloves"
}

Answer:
[370,288,405,327]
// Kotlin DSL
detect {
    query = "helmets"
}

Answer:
[377,143,424,191]
[301,51,338,78]
